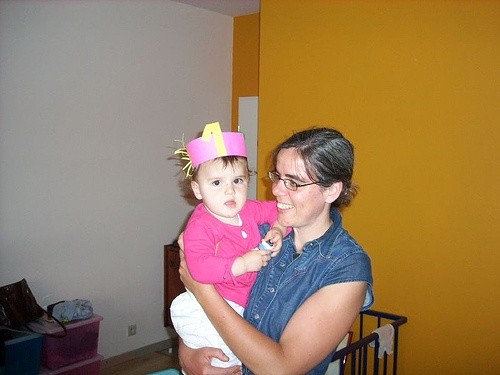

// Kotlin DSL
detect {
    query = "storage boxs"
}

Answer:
[0,307,104,375]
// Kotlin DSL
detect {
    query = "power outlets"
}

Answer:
[127,324,138,336]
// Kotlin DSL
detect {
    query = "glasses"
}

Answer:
[267,170,320,191]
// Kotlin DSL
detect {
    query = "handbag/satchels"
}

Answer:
[0,278,67,338]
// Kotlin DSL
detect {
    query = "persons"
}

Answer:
[168,120,292,375]
[176,127,375,375]
